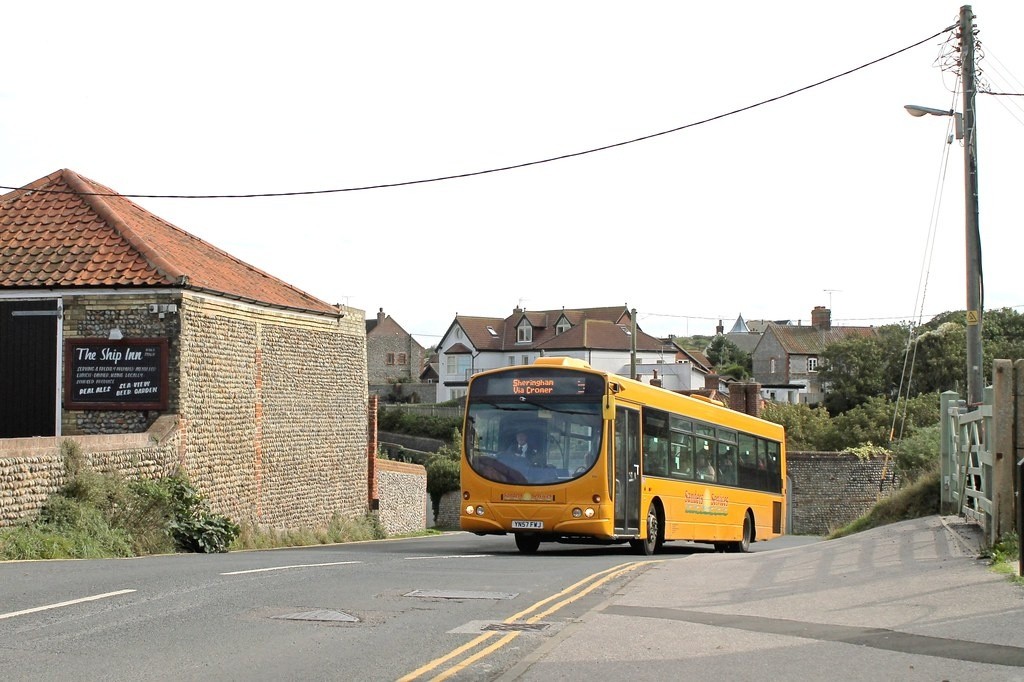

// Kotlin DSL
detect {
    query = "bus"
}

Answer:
[459,356,787,555]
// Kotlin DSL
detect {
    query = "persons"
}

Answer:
[507,432,538,465]
[695,449,770,491]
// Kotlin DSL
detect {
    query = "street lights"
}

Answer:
[903,102,987,504]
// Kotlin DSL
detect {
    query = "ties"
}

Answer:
[519,447,523,457]
